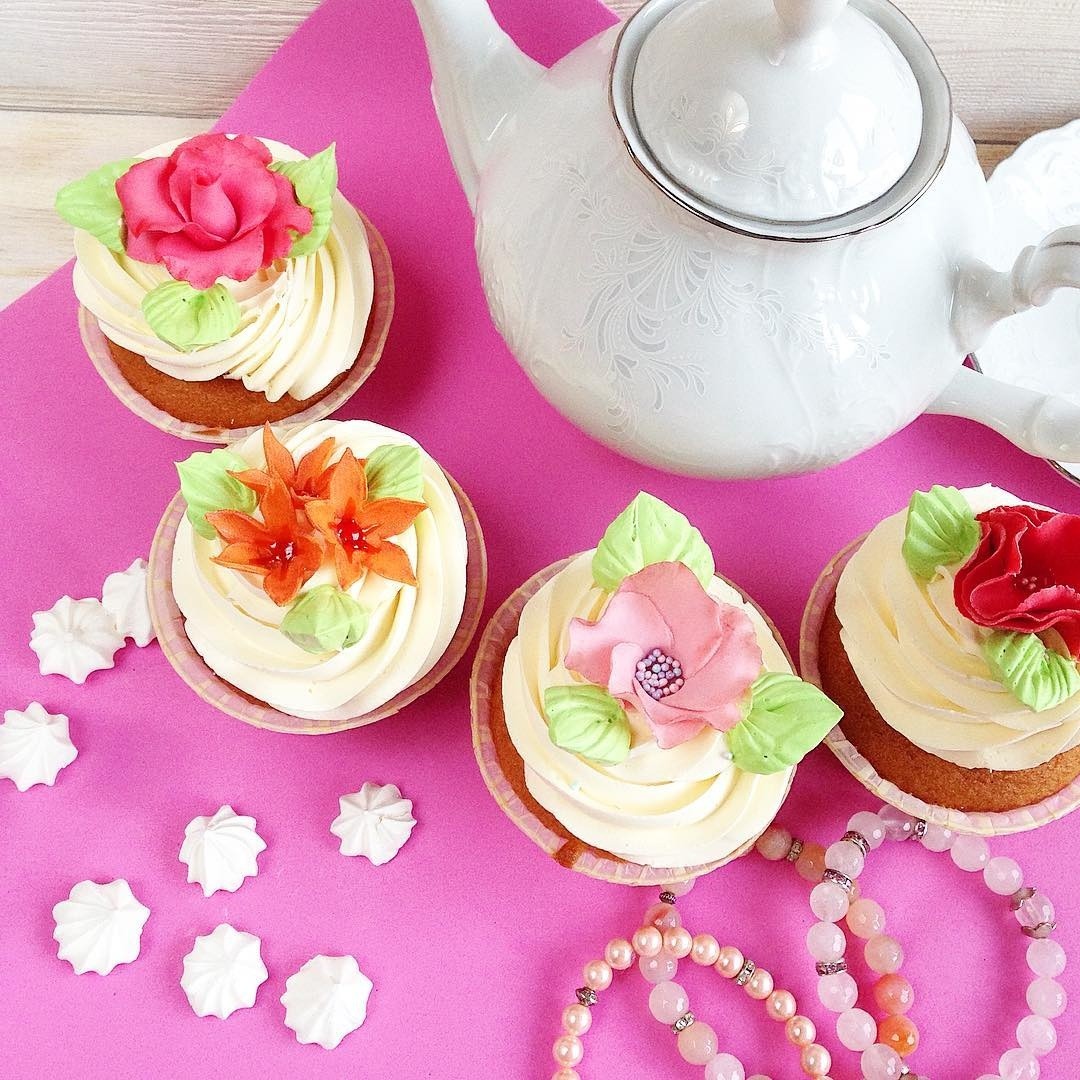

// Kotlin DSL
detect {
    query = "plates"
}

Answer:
[967,120,1080,485]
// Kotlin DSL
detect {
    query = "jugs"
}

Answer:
[408,0,1080,486]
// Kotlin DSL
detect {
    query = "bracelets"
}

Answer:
[551,803,1067,1080]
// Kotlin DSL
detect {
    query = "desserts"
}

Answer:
[144,420,487,734]
[54,129,395,443]
[467,491,846,886]
[799,483,1080,837]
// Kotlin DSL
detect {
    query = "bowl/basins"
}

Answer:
[145,460,488,735]
[469,547,799,888]
[797,532,1079,837]
[78,208,396,445]
